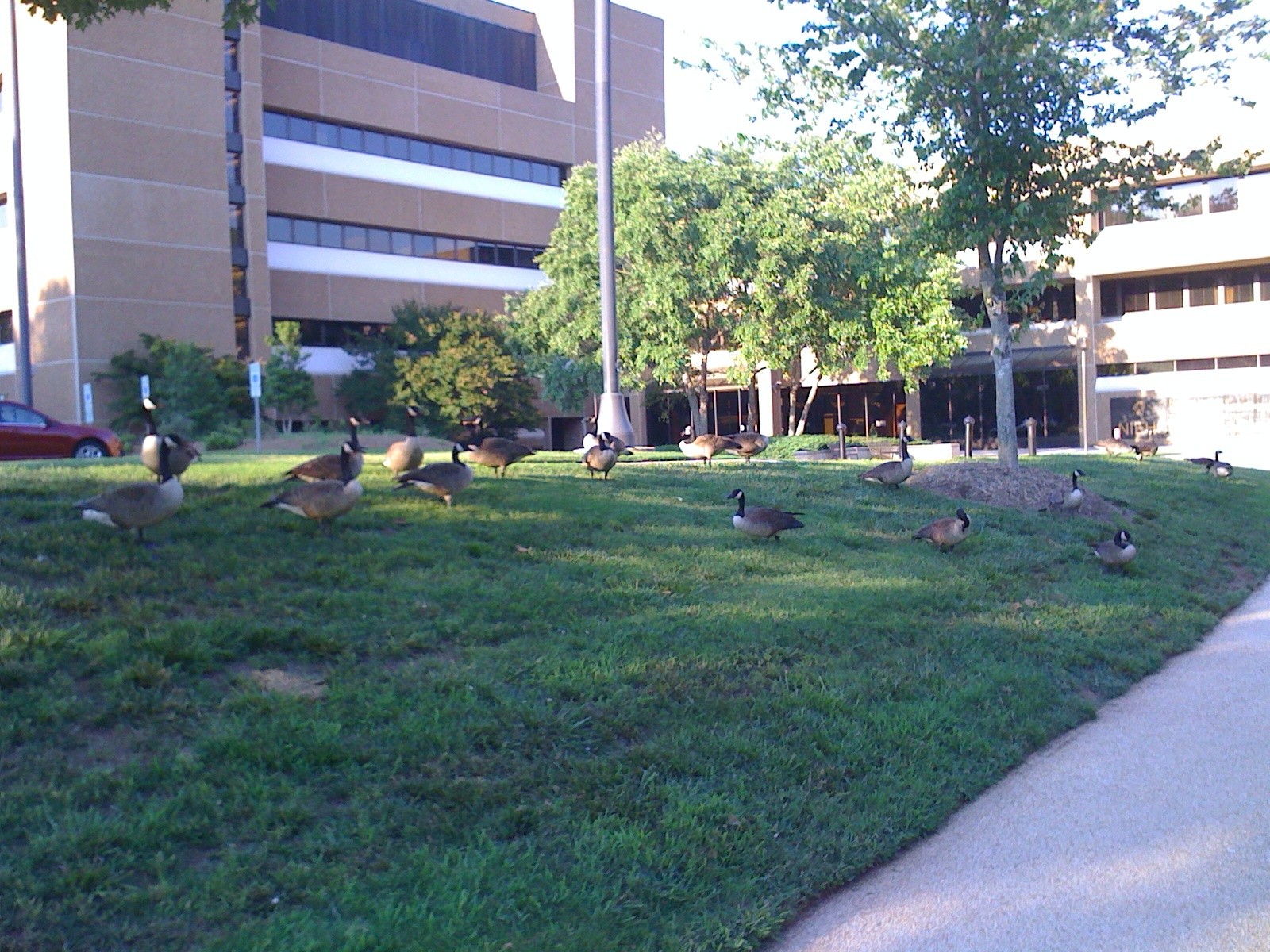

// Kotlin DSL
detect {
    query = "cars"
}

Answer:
[0,399,124,460]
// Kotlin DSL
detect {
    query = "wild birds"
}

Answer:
[581,414,634,456]
[136,395,200,484]
[1088,531,1135,570]
[1094,438,1142,462]
[1210,450,1232,483]
[381,406,424,476]
[282,414,371,485]
[461,414,537,478]
[390,441,476,507]
[678,425,741,470]
[259,441,368,532]
[858,435,917,489]
[724,432,768,462]
[1037,470,1087,513]
[724,489,806,544]
[582,432,617,480]
[70,434,194,544]
[911,507,972,553]
